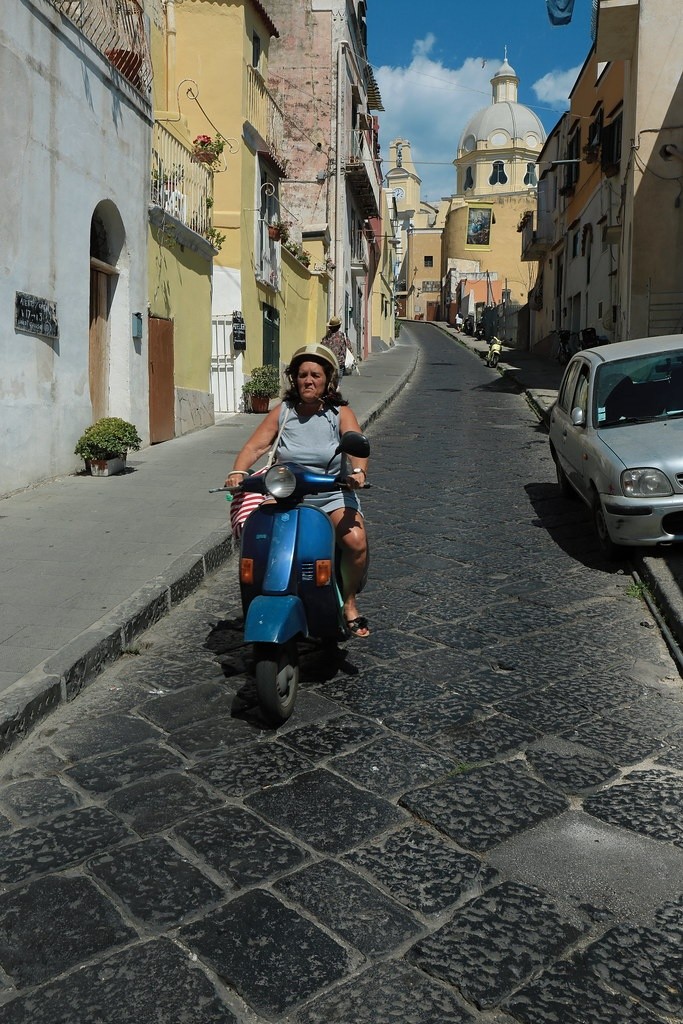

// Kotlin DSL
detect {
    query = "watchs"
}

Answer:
[351,467,367,481]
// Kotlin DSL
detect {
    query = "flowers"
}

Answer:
[193,133,226,170]
[297,251,312,265]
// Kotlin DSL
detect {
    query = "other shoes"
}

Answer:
[458,331,460,333]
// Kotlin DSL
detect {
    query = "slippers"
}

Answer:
[344,613,370,638]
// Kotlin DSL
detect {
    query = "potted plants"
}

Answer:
[166,174,177,192]
[241,364,282,414]
[73,415,142,477]
[269,220,293,242]
[559,183,575,197]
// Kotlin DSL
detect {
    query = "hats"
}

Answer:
[325,316,343,326]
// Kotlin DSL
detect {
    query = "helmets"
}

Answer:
[285,343,340,407]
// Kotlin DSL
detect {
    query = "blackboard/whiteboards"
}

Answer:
[14,289,60,339]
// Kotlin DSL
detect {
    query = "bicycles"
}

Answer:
[549,329,576,365]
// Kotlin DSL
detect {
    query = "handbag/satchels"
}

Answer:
[344,332,356,374]
[229,465,272,542]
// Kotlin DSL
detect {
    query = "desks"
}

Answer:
[164,189,187,225]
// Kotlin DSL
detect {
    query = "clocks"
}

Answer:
[393,187,405,202]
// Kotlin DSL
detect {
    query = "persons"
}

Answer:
[320,317,353,391]
[455,312,464,333]
[223,342,371,639]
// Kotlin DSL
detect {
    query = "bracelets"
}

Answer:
[228,469,251,478]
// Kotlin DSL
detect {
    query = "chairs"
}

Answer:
[599,373,633,419]
[663,367,683,410]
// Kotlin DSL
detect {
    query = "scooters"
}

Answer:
[208,431,374,724]
[464,315,473,335]
[485,336,512,369]
[476,316,484,340]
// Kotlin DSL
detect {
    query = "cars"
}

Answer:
[549,335,683,558]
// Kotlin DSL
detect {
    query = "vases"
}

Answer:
[303,263,309,268]
[194,153,215,162]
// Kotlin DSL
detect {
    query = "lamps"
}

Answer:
[374,234,401,244]
